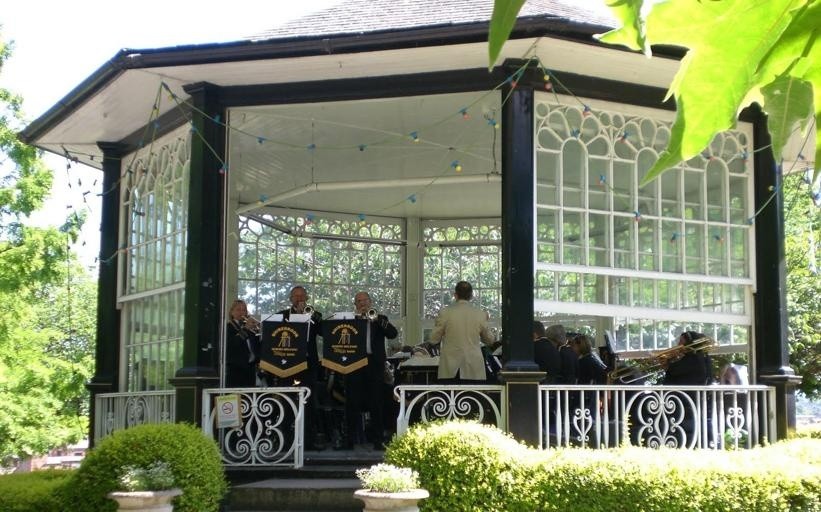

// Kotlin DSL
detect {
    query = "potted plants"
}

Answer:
[105,462,183,512]
[353,463,430,512]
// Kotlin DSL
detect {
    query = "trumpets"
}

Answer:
[240,315,260,336]
[363,307,377,320]
[298,302,314,316]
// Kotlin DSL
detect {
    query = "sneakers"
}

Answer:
[332,438,355,450]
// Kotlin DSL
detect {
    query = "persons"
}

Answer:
[333,291,399,451]
[610,361,643,412]
[532,320,607,423]
[224,299,261,450]
[269,286,325,451]
[428,281,497,418]
[635,331,713,421]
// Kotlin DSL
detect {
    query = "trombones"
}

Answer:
[608,337,713,384]
